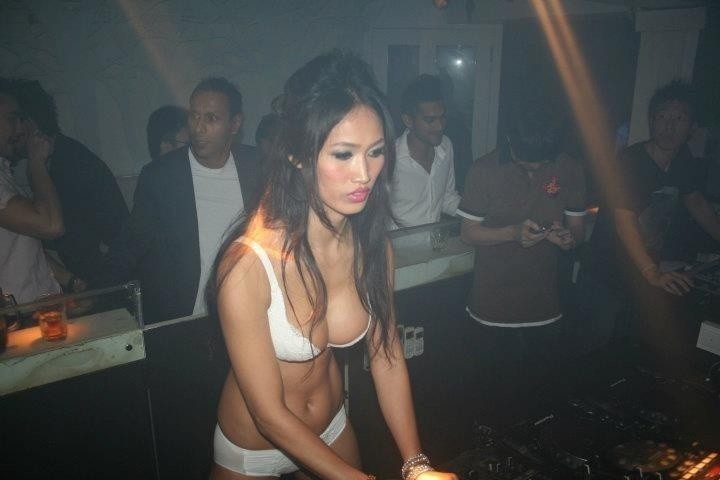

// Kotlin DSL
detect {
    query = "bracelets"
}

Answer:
[401,453,432,480]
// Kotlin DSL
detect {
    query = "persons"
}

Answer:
[387,76,463,231]
[205,50,456,480]
[454,96,590,393]
[2,77,274,324]
[580,81,720,356]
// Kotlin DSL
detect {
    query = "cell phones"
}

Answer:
[534,221,552,234]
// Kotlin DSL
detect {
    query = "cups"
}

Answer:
[34,293,67,341]
[431,230,449,252]
[0,287,22,353]
